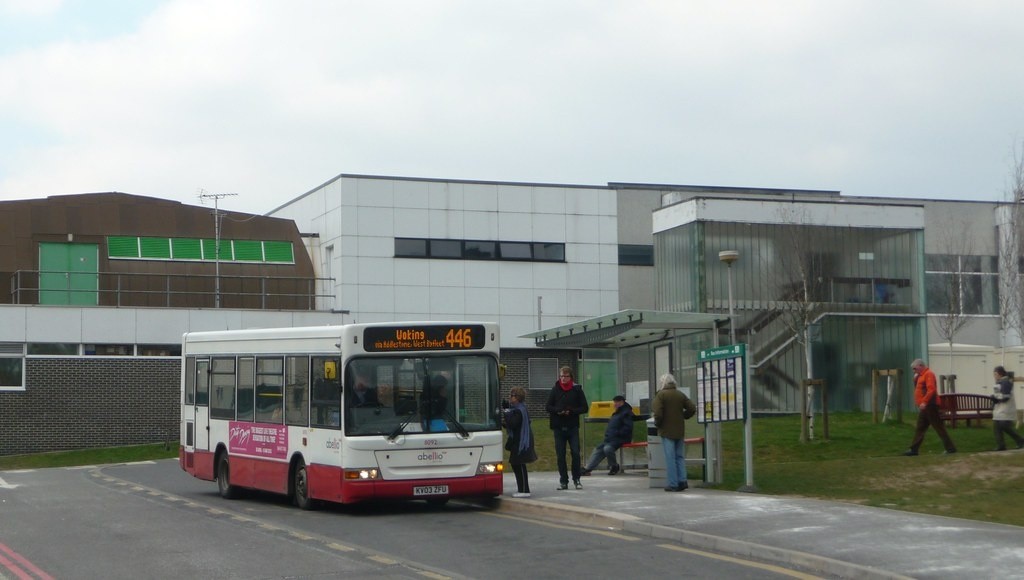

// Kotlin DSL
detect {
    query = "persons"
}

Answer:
[651,374,696,491]
[897,359,958,456]
[545,367,589,490]
[418,376,448,421]
[502,387,538,497]
[579,396,635,476]
[991,366,1024,450]
[347,372,384,409]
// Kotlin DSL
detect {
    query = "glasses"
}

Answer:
[509,394,518,398]
[560,375,570,377]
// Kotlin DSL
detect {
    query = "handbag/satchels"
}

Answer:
[505,436,513,450]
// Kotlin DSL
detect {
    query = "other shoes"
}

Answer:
[607,464,620,475]
[902,448,918,456]
[580,467,591,476]
[943,449,956,454]
[679,481,688,489]
[665,485,680,492]
[574,478,582,489]
[512,491,531,498]
[557,483,568,490]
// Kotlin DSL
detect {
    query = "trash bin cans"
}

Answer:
[646,417,667,488]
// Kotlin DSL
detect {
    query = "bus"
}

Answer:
[179,322,508,513]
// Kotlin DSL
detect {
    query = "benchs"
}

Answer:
[617,436,718,474]
[937,393,994,431]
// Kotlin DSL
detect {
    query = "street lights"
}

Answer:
[717,248,741,345]
